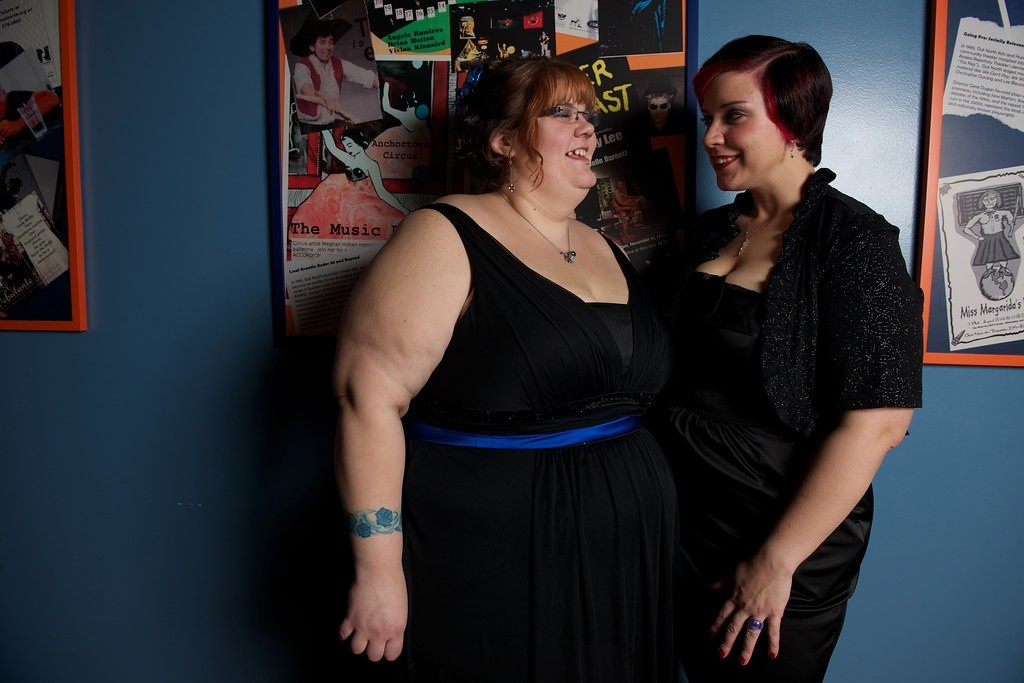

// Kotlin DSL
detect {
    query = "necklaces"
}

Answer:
[735,198,801,257]
[491,190,584,263]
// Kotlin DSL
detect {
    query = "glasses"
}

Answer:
[537,106,600,127]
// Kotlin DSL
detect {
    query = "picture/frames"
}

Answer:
[263,0,698,353]
[916,0,1024,368]
[0,1,87,332]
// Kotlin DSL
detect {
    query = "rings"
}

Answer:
[748,617,764,630]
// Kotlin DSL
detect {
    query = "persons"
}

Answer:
[292,28,380,137]
[539,30,550,57]
[655,35,923,683]
[333,57,680,682]
[609,181,640,239]
